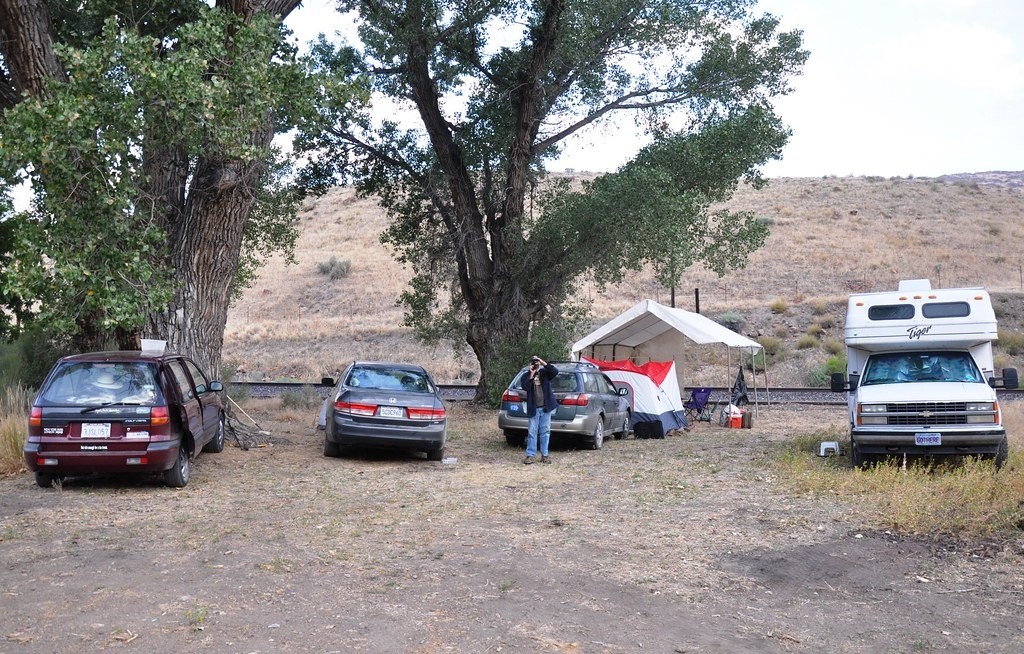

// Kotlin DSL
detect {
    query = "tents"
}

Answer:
[570,298,770,439]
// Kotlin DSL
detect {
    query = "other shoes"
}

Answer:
[540,457,552,465]
[524,456,535,464]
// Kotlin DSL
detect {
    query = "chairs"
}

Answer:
[682,387,713,425]
[400,376,418,390]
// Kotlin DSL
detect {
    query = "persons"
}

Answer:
[520,356,560,464]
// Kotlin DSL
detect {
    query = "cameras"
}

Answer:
[532,359,539,369]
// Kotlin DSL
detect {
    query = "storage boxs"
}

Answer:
[140,338,168,351]
[728,414,743,428]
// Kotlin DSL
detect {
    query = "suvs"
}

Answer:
[498,359,631,450]
[24,349,228,488]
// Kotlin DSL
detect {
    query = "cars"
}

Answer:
[321,360,448,462]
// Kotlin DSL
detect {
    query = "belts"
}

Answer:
[536,406,544,408]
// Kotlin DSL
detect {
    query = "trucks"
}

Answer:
[831,278,1019,472]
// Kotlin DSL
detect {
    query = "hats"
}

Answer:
[530,356,539,360]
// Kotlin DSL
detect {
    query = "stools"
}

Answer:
[819,440,840,455]
